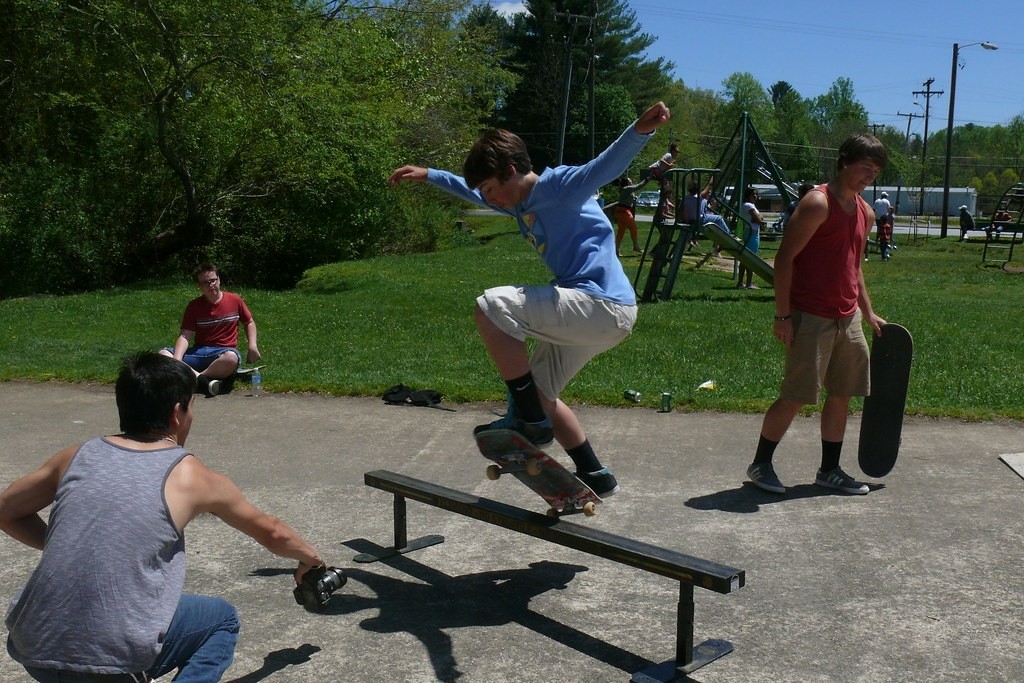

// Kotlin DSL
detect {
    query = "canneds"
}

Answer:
[624,389,643,402]
[661,392,671,412]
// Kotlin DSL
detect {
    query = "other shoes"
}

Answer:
[736,281,746,290]
[198,375,222,397]
[689,240,700,247]
[712,242,723,259]
[883,253,890,262]
[962,239,968,243]
[744,283,762,289]
[963,233,969,240]
[633,247,645,253]
[734,236,742,241]
[618,253,624,258]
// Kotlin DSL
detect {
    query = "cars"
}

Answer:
[634,190,661,209]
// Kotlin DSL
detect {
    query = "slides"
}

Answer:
[703,222,775,285]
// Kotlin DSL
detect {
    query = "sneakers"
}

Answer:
[745,459,789,493]
[569,464,621,500]
[816,465,872,495]
[471,403,555,450]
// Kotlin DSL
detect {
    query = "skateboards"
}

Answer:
[856,321,915,481]
[237,363,267,377]
[475,428,605,521]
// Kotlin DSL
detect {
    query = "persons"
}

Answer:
[958,205,975,239]
[981,212,1014,232]
[158,265,260,396]
[386,100,670,498]
[872,191,891,241]
[736,187,763,290]
[878,216,891,261]
[682,175,730,259]
[783,184,814,231]
[615,176,650,257]
[647,144,679,189]
[0,352,327,683]
[746,134,886,494]
[655,190,674,233]
[887,208,894,241]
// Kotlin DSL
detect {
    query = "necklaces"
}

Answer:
[140,436,175,443]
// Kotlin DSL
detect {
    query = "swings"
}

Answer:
[639,118,748,228]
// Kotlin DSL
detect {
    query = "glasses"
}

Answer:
[197,275,219,287]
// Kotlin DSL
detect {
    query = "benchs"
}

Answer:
[960,220,1024,243]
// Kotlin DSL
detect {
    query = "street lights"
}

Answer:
[940,40,1000,239]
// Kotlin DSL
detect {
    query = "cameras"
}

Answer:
[294,566,346,614]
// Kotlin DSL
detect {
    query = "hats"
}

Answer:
[958,205,967,210]
[881,191,889,197]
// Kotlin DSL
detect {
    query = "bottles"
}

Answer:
[251,367,261,396]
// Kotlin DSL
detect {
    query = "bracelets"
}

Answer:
[774,315,791,321]
[311,554,324,570]
